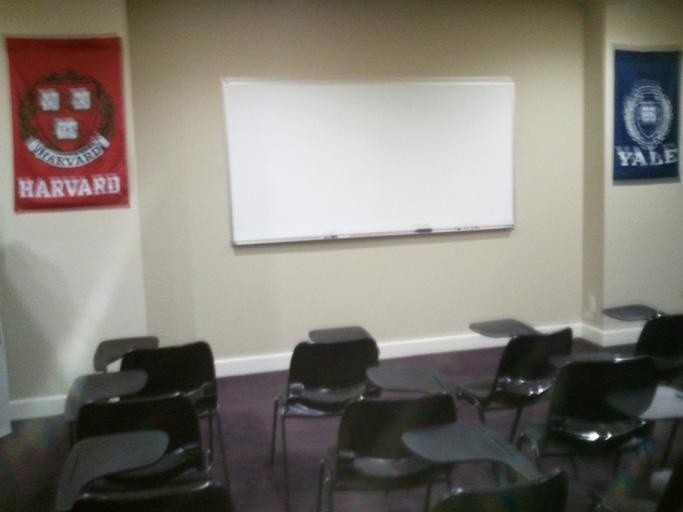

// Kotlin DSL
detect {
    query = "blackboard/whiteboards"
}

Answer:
[220,76,517,248]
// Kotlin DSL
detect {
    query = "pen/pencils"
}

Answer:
[416,228,432,232]
